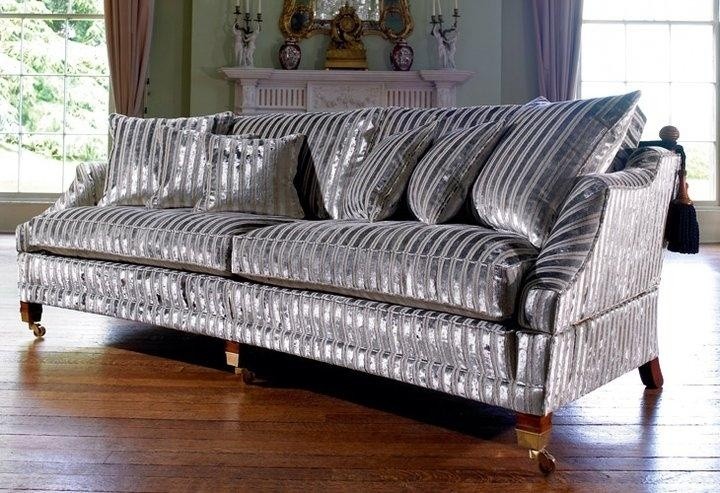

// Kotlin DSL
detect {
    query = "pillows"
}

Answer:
[94,111,304,217]
[466,91,642,252]
[401,118,509,226]
[342,116,444,222]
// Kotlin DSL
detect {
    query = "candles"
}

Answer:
[431,1,458,17]
[235,0,262,13]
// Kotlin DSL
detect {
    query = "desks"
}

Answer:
[215,68,476,113]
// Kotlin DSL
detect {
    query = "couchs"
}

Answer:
[15,105,664,476]
